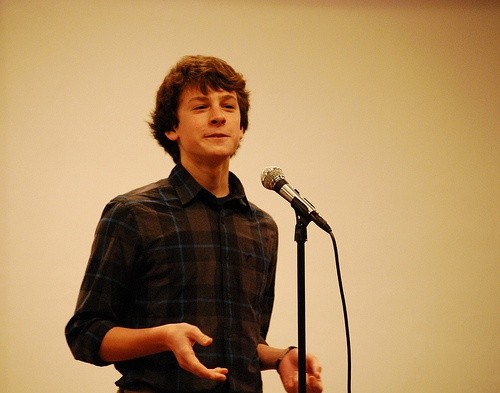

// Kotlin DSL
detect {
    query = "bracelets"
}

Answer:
[276,345,298,371]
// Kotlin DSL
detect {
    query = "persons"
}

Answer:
[63,51,325,393]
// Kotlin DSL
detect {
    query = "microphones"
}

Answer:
[261,166,332,234]
